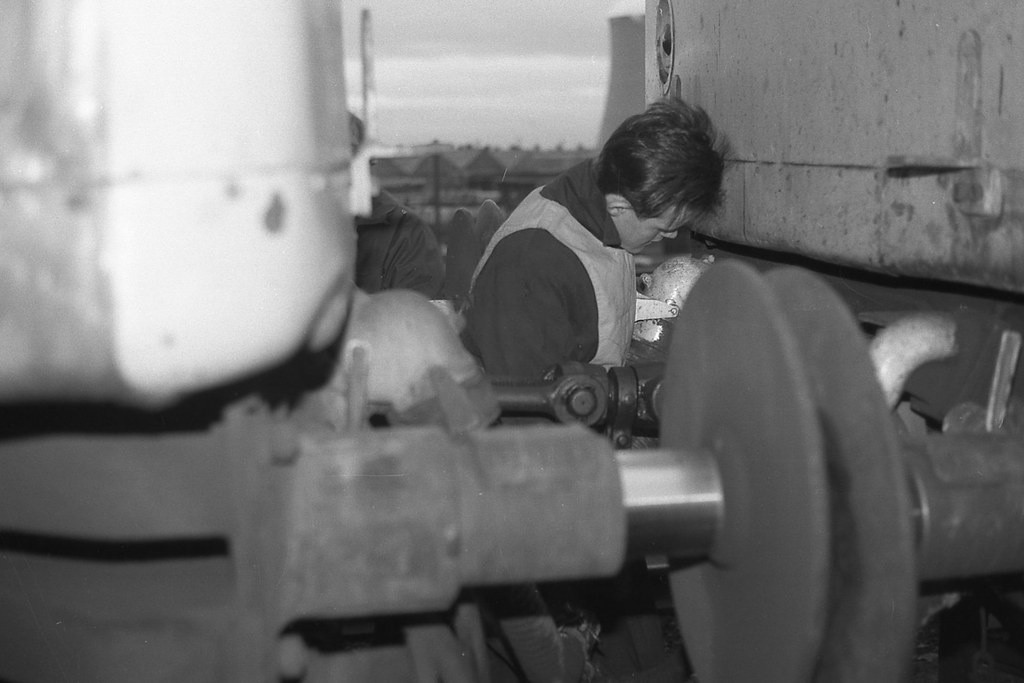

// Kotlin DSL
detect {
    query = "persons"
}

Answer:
[461,94,743,428]
[348,111,446,299]
